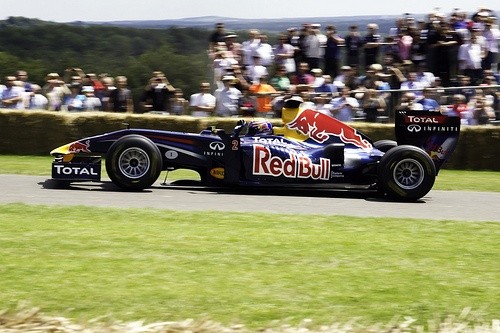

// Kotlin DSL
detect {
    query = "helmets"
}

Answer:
[248,120,272,136]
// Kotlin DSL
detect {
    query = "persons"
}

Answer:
[169,88,188,115]
[188,82,219,117]
[140,71,175,114]
[232,120,272,137]
[209,23,359,121]
[0,68,134,112]
[333,12,500,125]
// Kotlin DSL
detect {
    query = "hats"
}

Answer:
[365,63,383,73]
[470,23,482,30]
[43,72,62,82]
[340,65,351,70]
[223,30,237,39]
[309,23,321,26]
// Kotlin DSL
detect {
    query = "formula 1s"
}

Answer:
[50,92,462,204]
